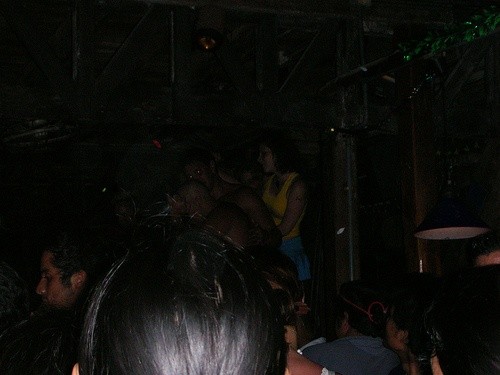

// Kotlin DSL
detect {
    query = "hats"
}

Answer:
[221,166,267,191]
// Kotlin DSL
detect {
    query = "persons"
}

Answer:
[0,126,443,375]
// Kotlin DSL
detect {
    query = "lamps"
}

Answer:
[410,54,490,239]
[190,5,227,53]
